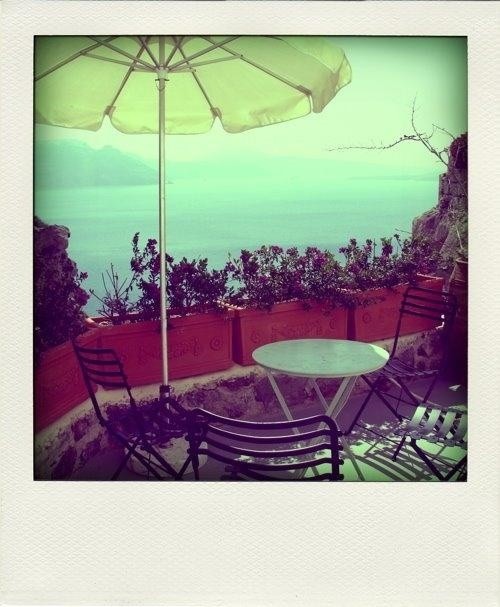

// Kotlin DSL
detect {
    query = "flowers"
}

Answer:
[99,234,227,328]
[33,216,94,352]
[226,238,339,310]
[329,237,452,296]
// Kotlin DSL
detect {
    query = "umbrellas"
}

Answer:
[33,35,355,475]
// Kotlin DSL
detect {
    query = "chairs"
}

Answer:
[68,338,203,479]
[347,287,459,436]
[179,407,344,480]
[394,399,467,481]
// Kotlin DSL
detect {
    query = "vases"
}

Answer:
[223,286,352,366]
[33,330,100,433]
[331,258,445,342]
[86,297,235,390]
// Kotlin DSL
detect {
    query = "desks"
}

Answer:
[251,338,391,480]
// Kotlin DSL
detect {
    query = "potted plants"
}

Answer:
[446,201,468,318]
